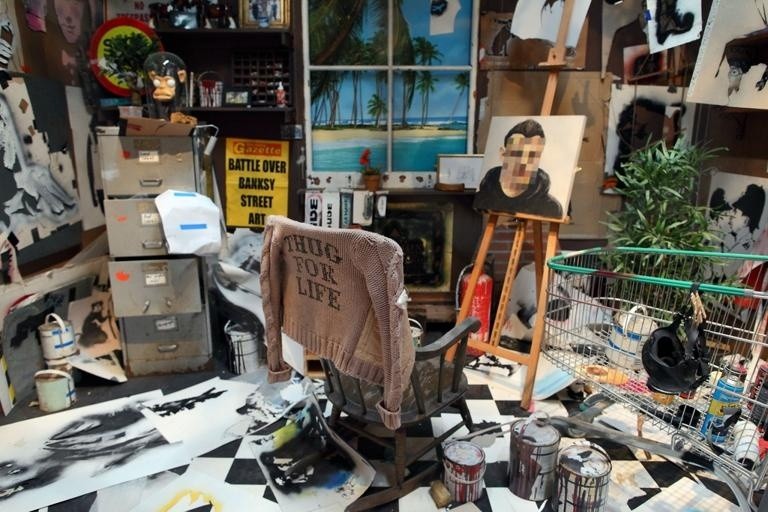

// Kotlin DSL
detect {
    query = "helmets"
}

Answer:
[641,281,710,398]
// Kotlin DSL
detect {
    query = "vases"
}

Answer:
[362,174,382,192]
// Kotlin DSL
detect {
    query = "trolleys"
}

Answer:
[511,237,768,511]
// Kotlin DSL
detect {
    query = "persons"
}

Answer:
[46,0,93,68]
[473,118,562,219]
[78,300,110,346]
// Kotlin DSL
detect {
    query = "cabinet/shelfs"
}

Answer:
[95,123,214,375]
[152,0,306,232]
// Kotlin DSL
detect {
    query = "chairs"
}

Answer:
[257,213,484,512]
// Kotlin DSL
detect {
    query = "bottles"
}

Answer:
[747,360,767,433]
[699,373,745,444]
[733,420,762,471]
[723,359,749,378]
[276,81,286,108]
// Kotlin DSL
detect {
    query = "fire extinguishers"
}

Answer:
[453,252,496,357]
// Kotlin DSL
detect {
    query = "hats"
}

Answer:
[731,183,766,231]
[709,188,732,220]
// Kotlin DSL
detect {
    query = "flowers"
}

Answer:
[358,148,383,176]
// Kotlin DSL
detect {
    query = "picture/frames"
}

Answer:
[237,0,291,29]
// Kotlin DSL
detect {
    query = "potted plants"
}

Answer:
[97,32,160,119]
